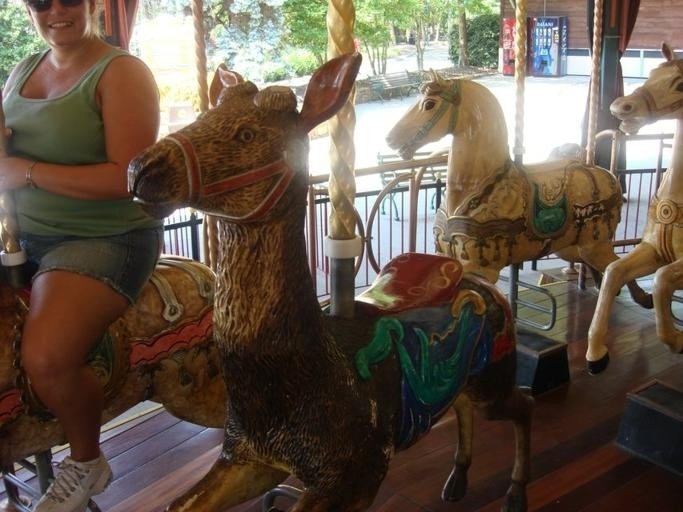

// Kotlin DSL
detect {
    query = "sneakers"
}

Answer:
[32,452,112,512]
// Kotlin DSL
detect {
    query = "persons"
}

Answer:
[0,0,164,512]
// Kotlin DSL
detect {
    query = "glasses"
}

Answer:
[26,0,81,11]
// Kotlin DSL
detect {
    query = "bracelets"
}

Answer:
[26,160,38,185]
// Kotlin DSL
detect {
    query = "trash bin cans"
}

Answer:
[501,15,568,76]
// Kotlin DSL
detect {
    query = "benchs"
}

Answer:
[356,67,477,102]
